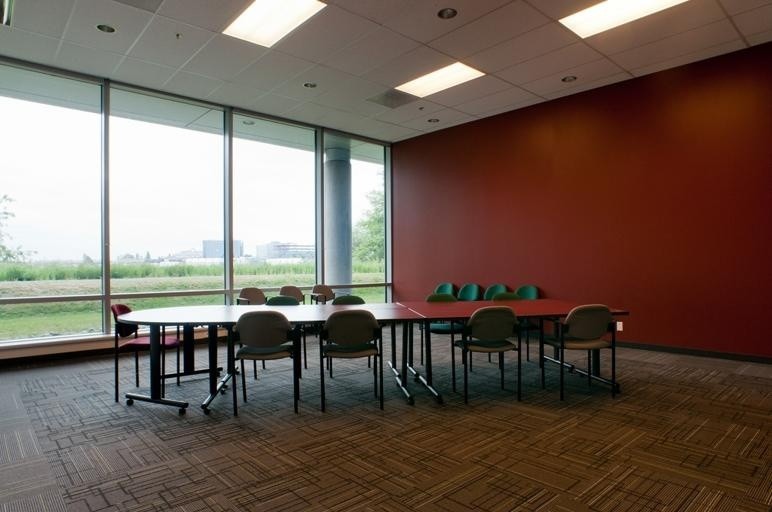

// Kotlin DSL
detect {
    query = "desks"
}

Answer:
[116,304,425,416]
[395,299,628,399]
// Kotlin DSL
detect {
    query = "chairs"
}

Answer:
[109,302,183,402]
[449,303,522,404]
[228,310,302,415]
[456,283,482,302]
[311,284,335,305]
[482,283,510,301]
[514,284,540,301]
[481,293,532,366]
[537,303,621,402]
[325,294,373,378]
[252,296,308,379]
[420,294,473,374]
[236,287,269,306]
[279,284,307,306]
[316,309,384,413]
[434,283,458,299]
